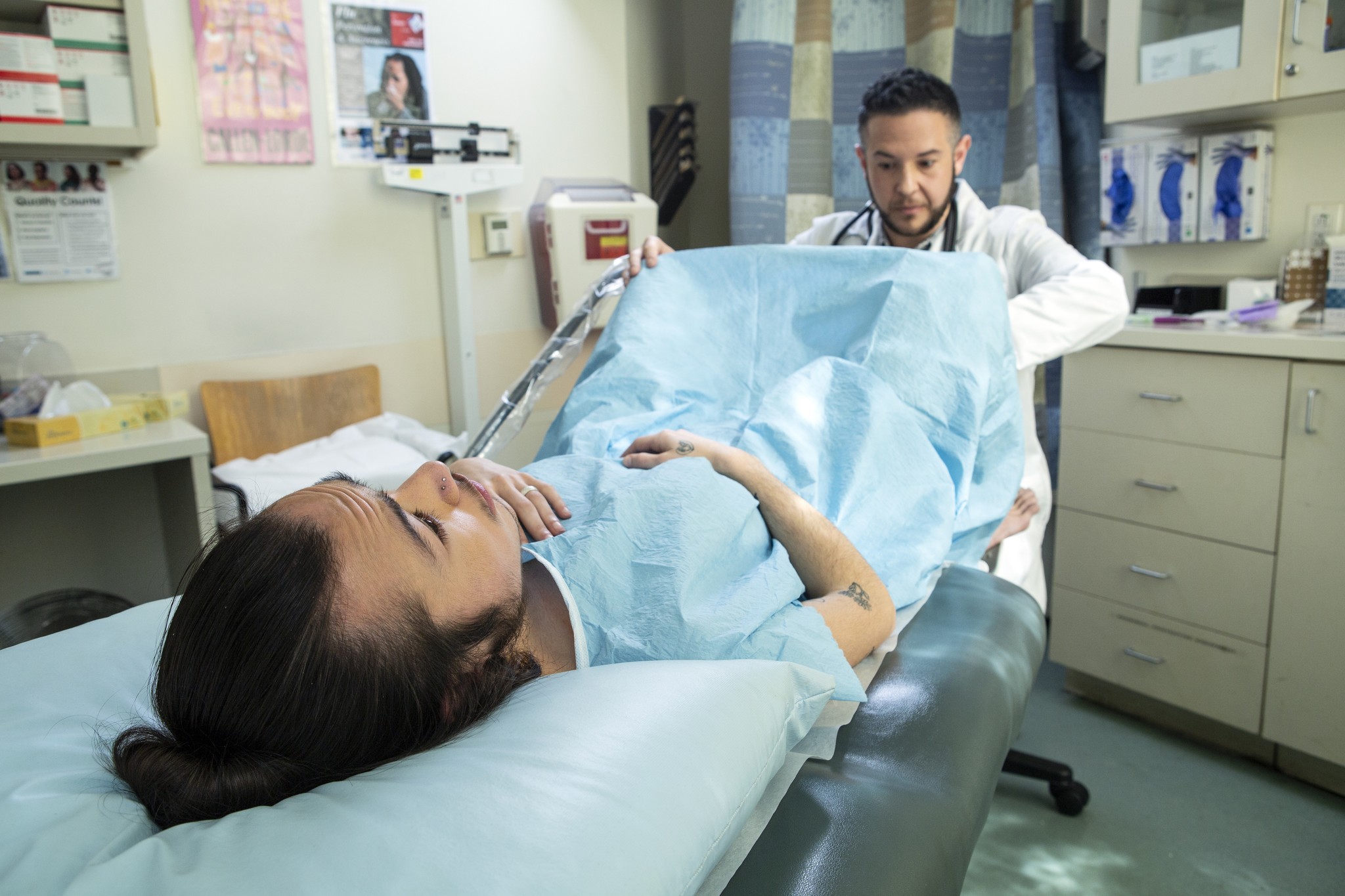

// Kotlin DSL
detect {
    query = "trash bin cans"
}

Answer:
[0,589,134,649]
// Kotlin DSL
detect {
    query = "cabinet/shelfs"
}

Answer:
[0,0,161,164]
[1097,0,1345,130]
[1038,347,1345,798]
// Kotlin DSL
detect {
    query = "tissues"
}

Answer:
[6,379,147,450]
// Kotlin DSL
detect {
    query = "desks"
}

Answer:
[0,401,220,597]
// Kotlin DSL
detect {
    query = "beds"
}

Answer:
[710,549,1055,896]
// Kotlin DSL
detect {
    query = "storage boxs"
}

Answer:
[42,6,127,52]
[1198,122,1278,244]
[6,388,172,452]
[55,47,128,87]
[1096,139,1146,249]
[61,87,87,124]
[0,33,59,81]
[1143,132,1204,247]
[0,76,64,123]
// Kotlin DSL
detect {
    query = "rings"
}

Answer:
[519,485,538,496]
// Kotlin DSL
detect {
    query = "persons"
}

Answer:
[621,67,1128,618]
[110,247,1042,833]
[367,53,432,162]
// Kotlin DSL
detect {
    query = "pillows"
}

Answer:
[0,590,837,896]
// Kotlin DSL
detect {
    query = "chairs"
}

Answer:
[199,363,382,532]
